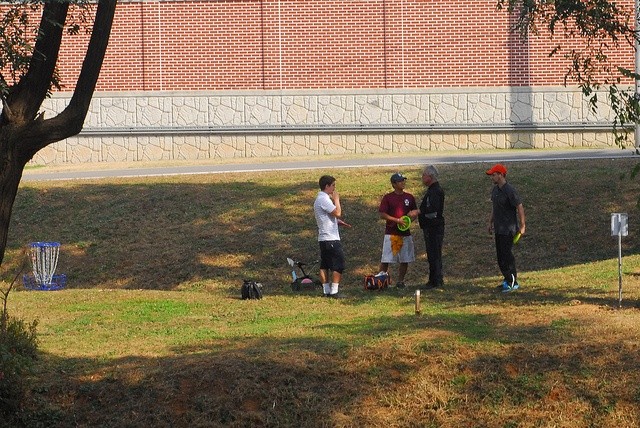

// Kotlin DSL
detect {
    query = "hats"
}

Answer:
[390,173,407,183]
[486,164,506,175]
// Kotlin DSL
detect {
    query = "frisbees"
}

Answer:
[397,216,411,231]
[513,232,521,244]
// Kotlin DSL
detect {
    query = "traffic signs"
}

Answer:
[610,213,628,237]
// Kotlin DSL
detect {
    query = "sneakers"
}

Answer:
[329,292,347,299]
[397,282,409,291]
[497,281,519,292]
[375,271,388,277]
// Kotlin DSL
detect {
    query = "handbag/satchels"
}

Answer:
[242,280,263,300]
[365,271,393,290]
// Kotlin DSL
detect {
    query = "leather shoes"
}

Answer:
[421,280,441,289]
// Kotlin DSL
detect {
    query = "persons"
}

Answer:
[486,164,526,294]
[408,165,445,289]
[379,173,417,288]
[313,176,347,299]
